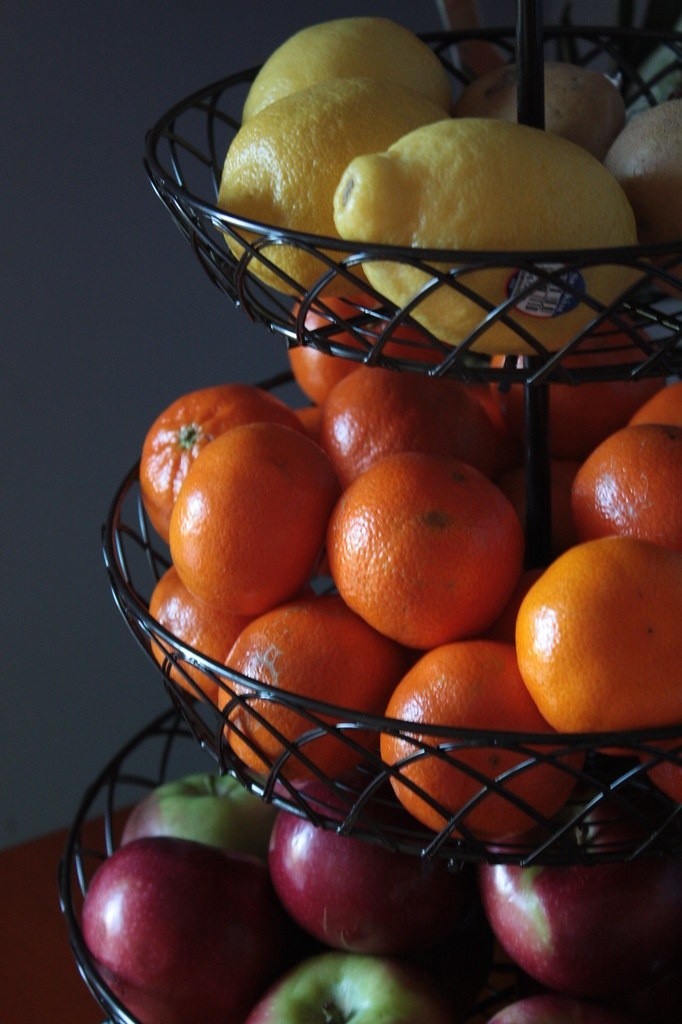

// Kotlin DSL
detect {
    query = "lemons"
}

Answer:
[214,20,682,359]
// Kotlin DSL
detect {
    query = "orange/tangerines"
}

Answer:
[137,292,682,842]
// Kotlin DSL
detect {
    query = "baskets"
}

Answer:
[141,25,682,385]
[54,700,682,1024]
[96,370,682,866]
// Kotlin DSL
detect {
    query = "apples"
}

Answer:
[79,767,682,1024]
